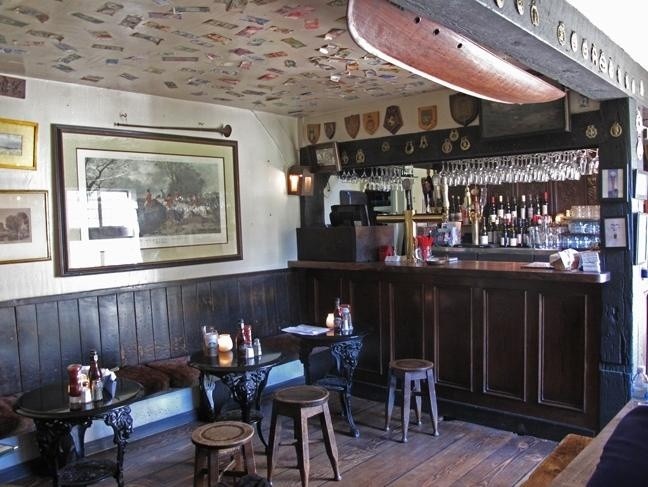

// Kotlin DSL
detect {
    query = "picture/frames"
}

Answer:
[0,117,38,170]
[0,190,52,264]
[307,141,341,172]
[49,123,243,276]
[601,169,648,266]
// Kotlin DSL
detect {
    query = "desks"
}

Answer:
[12,378,145,487]
[187,350,283,455]
[287,330,369,437]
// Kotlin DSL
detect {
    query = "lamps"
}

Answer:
[287,165,315,197]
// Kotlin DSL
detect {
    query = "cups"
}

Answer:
[413,245,431,261]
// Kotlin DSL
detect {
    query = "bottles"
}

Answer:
[199,318,263,359]
[384,243,392,256]
[450,193,600,249]
[325,296,354,336]
[66,347,114,404]
[631,366,648,407]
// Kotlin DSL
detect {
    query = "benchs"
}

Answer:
[0,334,328,484]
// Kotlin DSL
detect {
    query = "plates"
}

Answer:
[385,255,407,262]
[425,259,447,264]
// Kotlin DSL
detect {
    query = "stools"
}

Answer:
[385,359,439,442]
[191,421,256,487]
[267,386,343,487]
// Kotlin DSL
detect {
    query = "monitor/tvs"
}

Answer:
[331,204,370,226]
[479,91,571,144]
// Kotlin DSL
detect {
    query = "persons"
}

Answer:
[144,187,181,210]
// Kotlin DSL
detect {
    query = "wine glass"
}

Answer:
[337,146,599,191]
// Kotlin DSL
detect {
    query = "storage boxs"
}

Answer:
[296,224,394,262]
